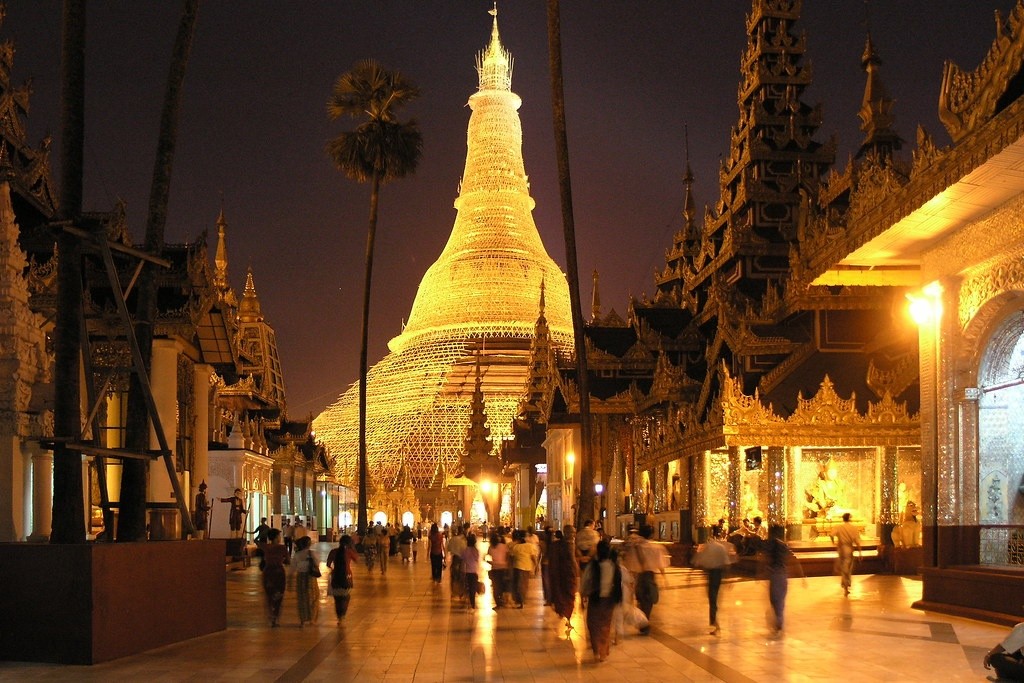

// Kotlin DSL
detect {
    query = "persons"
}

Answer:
[245,515,302,565]
[306,625,307,628]
[538,518,670,665]
[830,512,863,597]
[215,487,249,540]
[254,528,360,627]
[194,479,214,540]
[682,517,810,642]
[984,621,1024,683]
[350,516,546,610]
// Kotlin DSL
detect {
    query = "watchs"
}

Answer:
[987,649,993,657]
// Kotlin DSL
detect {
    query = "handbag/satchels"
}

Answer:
[443,558,446,568]
[623,607,650,630]
[308,550,321,578]
[476,582,484,594]
